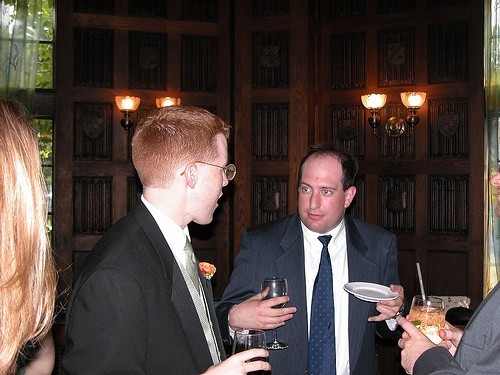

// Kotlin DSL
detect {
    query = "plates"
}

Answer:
[343,282,399,303]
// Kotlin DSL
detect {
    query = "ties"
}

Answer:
[308,234,336,375]
[183,235,201,295]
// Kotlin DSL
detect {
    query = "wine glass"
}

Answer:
[260,278,290,350]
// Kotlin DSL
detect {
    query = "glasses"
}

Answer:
[181,161,237,181]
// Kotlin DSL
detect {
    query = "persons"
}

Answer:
[59,105,272,375]
[214,146,408,375]
[0,96,58,375]
[395,157,500,375]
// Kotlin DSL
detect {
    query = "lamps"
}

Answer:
[360,92,427,136]
[115,95,181,129]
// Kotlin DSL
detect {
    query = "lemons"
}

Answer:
[411,320,424,329]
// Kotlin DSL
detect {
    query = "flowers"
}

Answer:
[198,261,217,281]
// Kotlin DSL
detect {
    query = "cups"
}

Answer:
[232,328,270,375]
[407,295,446,344]
